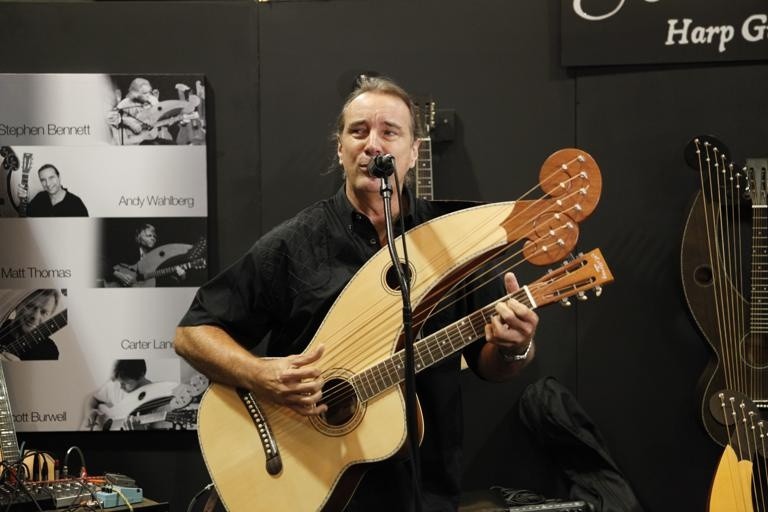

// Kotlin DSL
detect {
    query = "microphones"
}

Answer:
[368,154,392,177]
[143,98,151,104]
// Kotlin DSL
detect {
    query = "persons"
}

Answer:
[84,359,152,431]
[171,75,539,512]
[105,77,172,145]
[102,223,186,287]
[17,165,89,217]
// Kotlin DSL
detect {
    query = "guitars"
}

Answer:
[196,148,615,510]
[82,370,210,430]
[0,146,33,217]
[681,138,768,510]
[102,238,206,286]
[0,364,56,481]
[111,95,200,144]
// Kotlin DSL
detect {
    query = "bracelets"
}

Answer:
[501,338,533,361]
